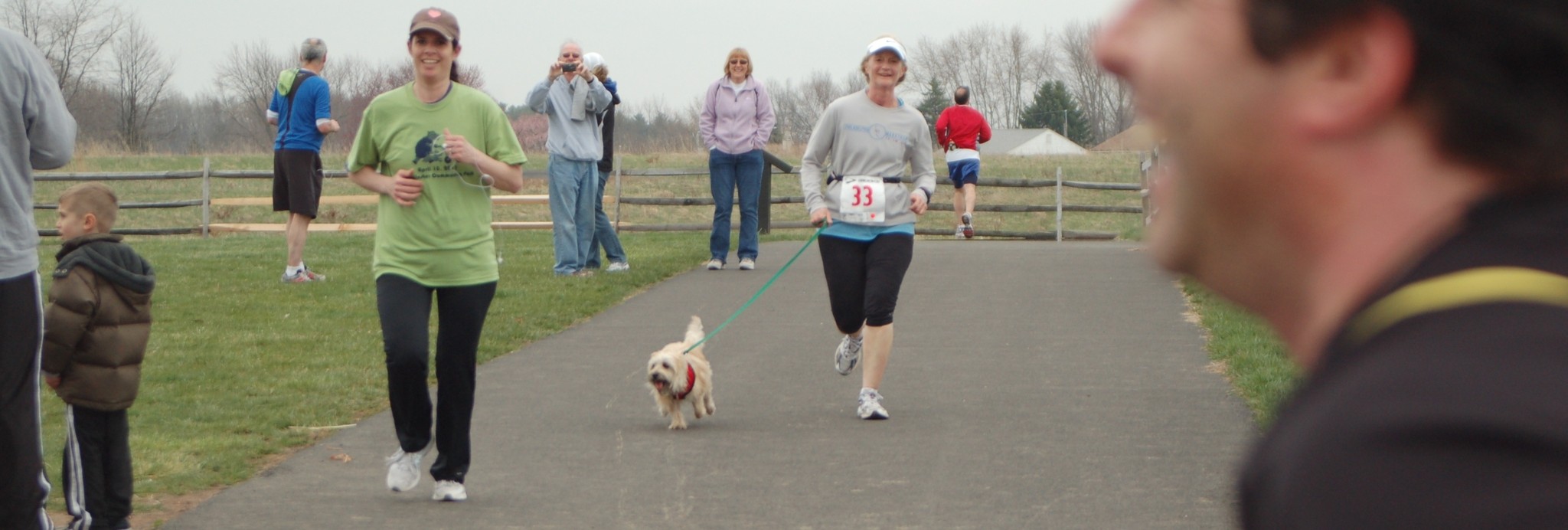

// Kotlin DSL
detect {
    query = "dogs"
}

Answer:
[646,316,717,430]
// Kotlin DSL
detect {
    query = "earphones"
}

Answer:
[442,143,449,150]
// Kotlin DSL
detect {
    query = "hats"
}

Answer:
[409,7,460,42]
[866,37,907,61]
[582,52,604,73]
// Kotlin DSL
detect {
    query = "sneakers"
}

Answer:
[385,431,435,493]
[432,480,467,501]
[856,388,890,419]
[605,261,630,272]
[833,326,864,375]
[961,212,973,238]
[955,225,965,237]
[280,264,326,283]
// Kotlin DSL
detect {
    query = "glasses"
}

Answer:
[562,53,580,58]
[730,59,748,64]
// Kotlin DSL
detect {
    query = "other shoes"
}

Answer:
[571,270,593,277]
[739,257,755,269]
[707,258,726,270]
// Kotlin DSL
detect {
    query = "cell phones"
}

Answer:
[562,61,577,73]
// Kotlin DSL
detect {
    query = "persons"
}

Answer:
[1089,1,1568,529]
[526,43,630,277]
[345,8,528,501]
[936,86,991,238]
[698,48,776,270]
[40,182,155,530]
[0,26,78,530]
[266,38,340,281]
[800,37,936,420]
